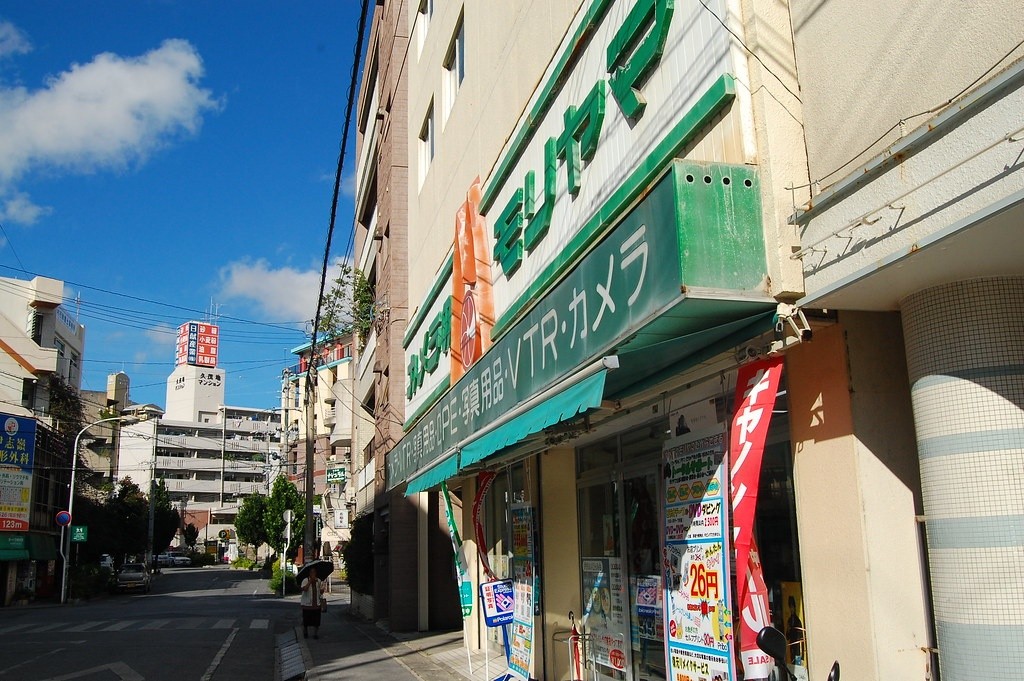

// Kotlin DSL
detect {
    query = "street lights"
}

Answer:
[64,415,139,603]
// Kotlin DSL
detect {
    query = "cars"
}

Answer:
[116,563,150,594]
[152,555,170,565]
[166,551,191,567]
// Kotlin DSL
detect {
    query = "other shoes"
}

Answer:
[303,630,309,639]
[312,633,318,639]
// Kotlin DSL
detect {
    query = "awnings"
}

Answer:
[405,307,777,499]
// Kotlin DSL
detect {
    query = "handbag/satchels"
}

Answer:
[320,598,327,612]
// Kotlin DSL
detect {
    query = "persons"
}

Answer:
[300,568,324,639]
[786,596,804,665]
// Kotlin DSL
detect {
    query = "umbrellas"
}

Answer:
[297,559,334,584]
[569,610,580,681]
